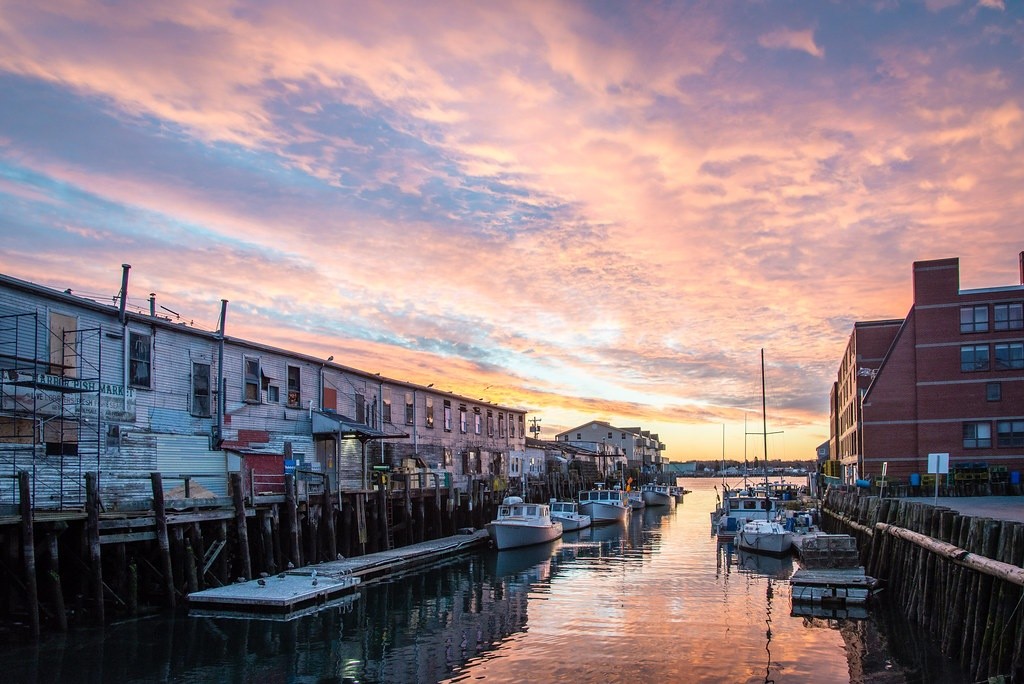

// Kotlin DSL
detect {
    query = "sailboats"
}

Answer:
[733,347,792,554]
[736,549,793,684]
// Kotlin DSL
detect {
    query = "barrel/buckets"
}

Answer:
[1010,471,1019,484]
[910,473,920,485]
[856,479,870,488]
[727,518,736,531]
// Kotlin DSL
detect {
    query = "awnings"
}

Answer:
[311,410,389,437]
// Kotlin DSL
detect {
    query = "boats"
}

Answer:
[639,476,675,507]
[544,497,592,532]
[710,413,783,542]
[578,482,633,524]
[484,473,563,550]
[670,487,683,504]
[625,486,646,510]
